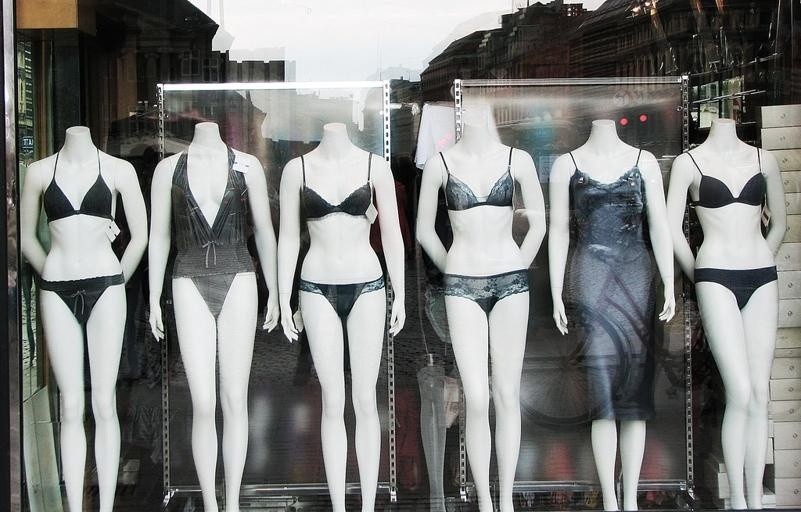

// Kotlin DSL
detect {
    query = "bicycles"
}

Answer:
[519,243,710,429]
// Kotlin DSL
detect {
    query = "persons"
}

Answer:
[664,117,789,509]
[548,119,678,511]
[415,114,548,511]
[18,126,148,512]
[274,122,408,512]
[148,122,281,512]
